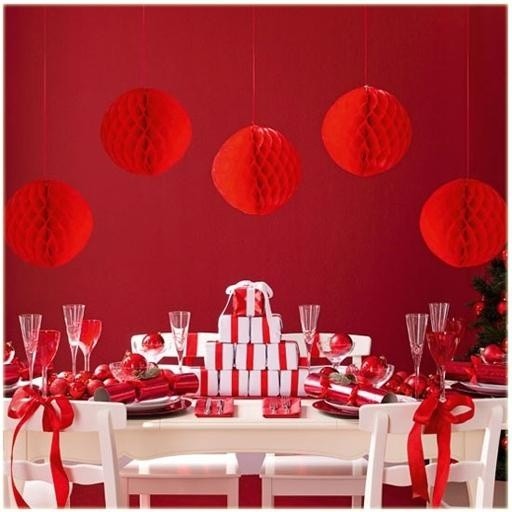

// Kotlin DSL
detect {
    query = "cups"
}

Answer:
[18,313,42,392]
[29,330,61,398]
[316,339,356,372]
[133,338,170,366]
[429,302,451,332]
[425,331,461,403]
[78,319,102,371]
[168,310,191,374]
[299,304,321,374]
[61,304,86,376]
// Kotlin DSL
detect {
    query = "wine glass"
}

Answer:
[405,313,429,402]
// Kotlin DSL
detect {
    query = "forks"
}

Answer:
[269,396,290,415]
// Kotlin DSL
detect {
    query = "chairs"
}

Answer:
[5,333,507,508]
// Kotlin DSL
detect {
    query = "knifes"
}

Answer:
[205,398,223,416]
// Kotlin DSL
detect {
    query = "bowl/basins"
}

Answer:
[109,361,152,382]
[349,363,395,389]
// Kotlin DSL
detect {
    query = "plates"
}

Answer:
[87,395,183,412]
[450,381,507,398]
[312,399,359,419]
[323,394,419,413]
[458,380,507,394]
[127,398,192,418]
